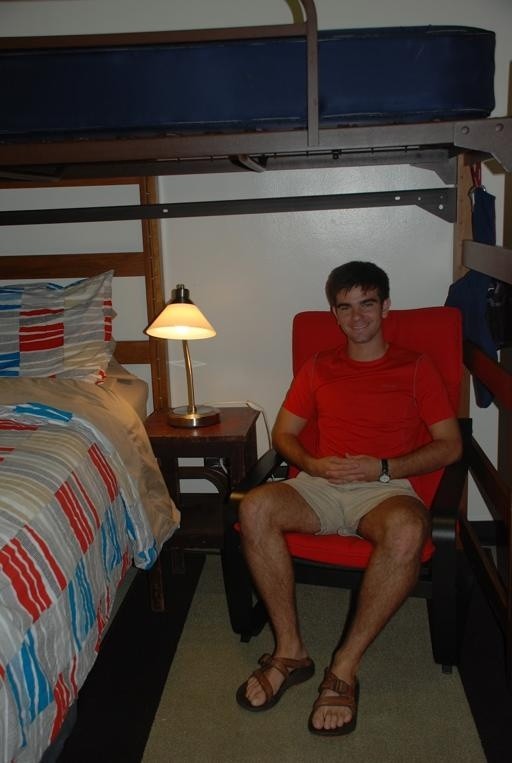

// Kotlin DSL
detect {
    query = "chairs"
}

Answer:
[220,306,471,675]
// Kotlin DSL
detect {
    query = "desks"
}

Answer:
[142,407,260,613]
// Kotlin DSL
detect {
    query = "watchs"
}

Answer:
[379,458,391,483]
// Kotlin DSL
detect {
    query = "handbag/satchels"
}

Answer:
[429,551,485,667]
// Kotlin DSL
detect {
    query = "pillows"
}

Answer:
[0,269,118,386]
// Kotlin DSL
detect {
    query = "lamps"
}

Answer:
[145,283,217,428]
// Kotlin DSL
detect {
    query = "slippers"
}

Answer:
[308,667,359,736]
[236,654,314,712]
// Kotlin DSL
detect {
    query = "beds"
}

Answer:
[0,176,172,763]
[0,0,512,690]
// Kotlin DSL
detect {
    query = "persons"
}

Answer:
[235,261,463,737]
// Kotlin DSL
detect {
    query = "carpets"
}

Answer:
[140,554,487,763]
